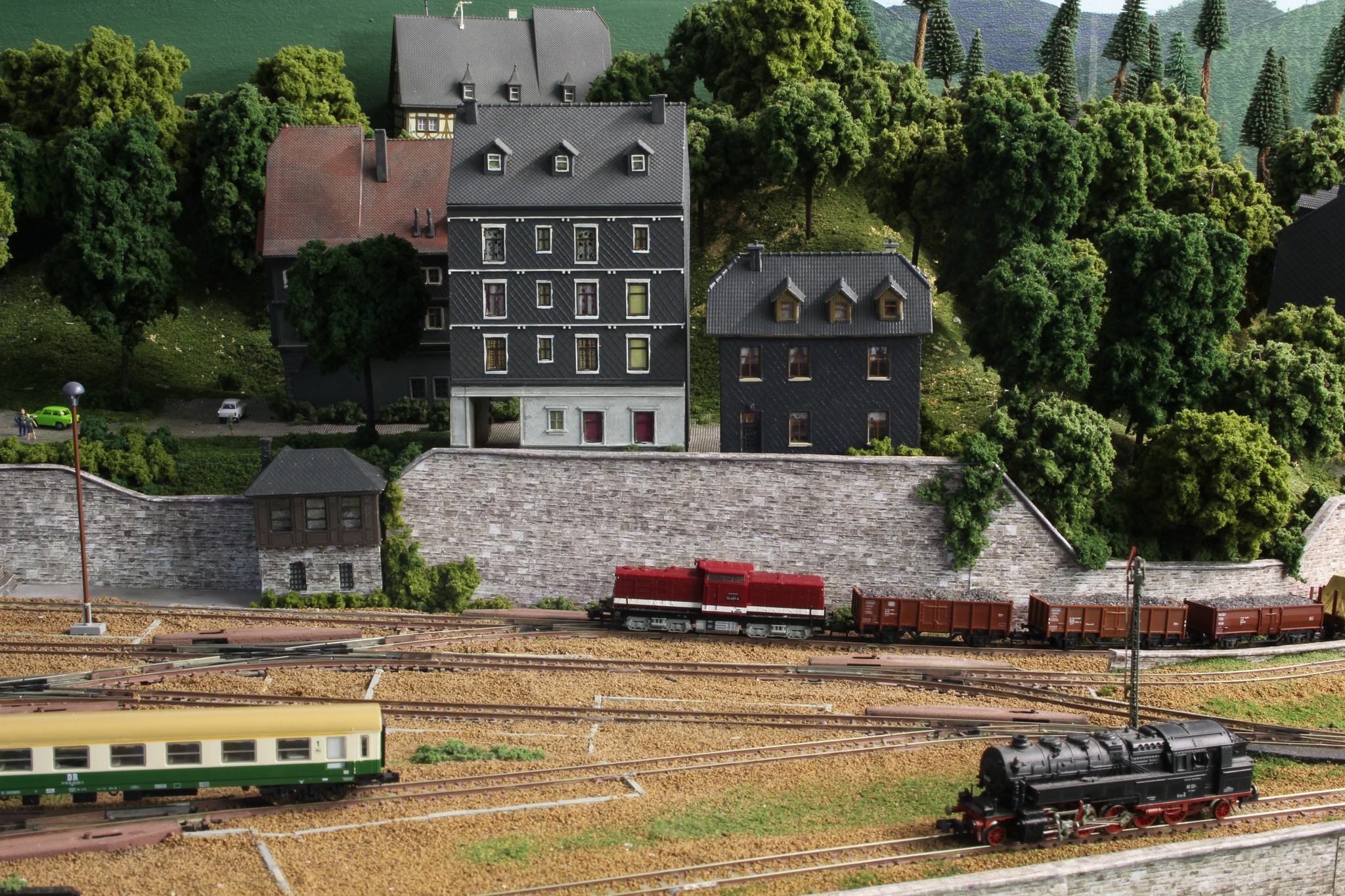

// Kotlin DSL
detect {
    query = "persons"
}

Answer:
[14,408,38,437]
[24,413,37,439]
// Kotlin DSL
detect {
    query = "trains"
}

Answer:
[945,720,1259,847]
[587,558,1325,650]
[0,704,386,805]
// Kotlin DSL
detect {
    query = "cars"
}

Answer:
[217,399,247,422]
[24,406,80,431]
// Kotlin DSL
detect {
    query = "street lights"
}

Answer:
[60,382,106,635]
